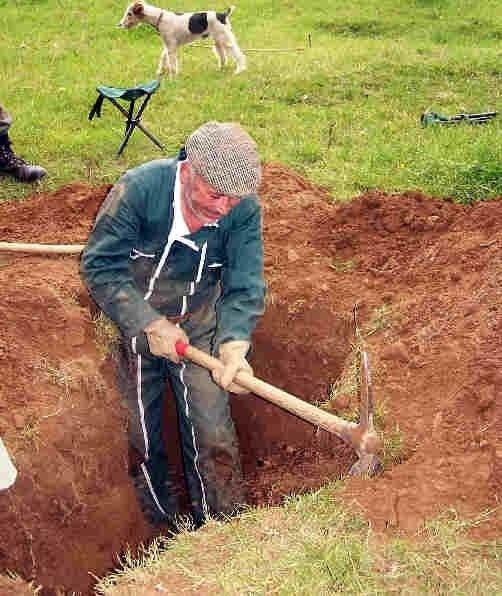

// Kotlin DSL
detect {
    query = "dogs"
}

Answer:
[119,0,247,79]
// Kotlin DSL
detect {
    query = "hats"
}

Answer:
[185,120,265,197]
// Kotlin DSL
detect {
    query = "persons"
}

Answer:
[77,120,268,536]
[0,107,49,184]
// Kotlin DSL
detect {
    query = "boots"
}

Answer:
[0,137,52,184]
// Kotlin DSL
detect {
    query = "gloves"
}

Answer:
[145,315,192,367]
[210,342,254,398]
